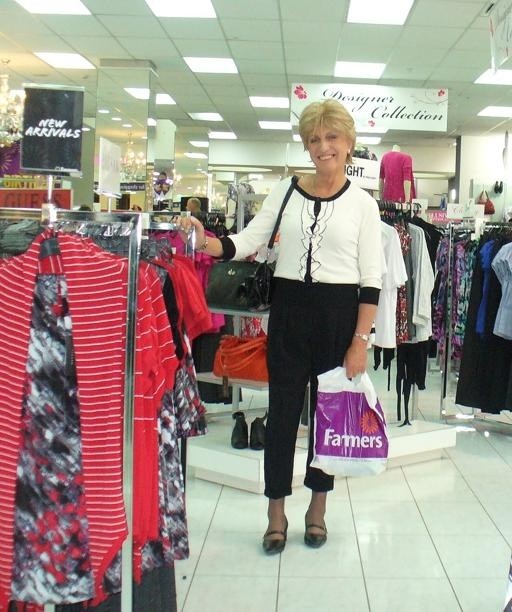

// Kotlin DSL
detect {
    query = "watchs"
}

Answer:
[354,332,369,342]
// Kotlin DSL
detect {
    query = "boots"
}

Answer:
[232,411,248,448]
[250,418,265,451]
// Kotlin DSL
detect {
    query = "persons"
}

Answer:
[378,143,416,203]
[174,98,387,555]
[186,197,202,213]
[152,172,172,204]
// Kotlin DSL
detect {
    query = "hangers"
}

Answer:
[34,208,200,259]
[376,202,512,249]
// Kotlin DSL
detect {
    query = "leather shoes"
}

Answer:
[304,516,327,548]
[495,181,503,193]
[263,516,287,555]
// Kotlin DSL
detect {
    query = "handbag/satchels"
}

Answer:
[478,200,495,214]
[214,334,271,381]
[205,261,258,309]
[235,264,274,312]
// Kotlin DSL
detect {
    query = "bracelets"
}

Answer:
[197,238,208,252]
